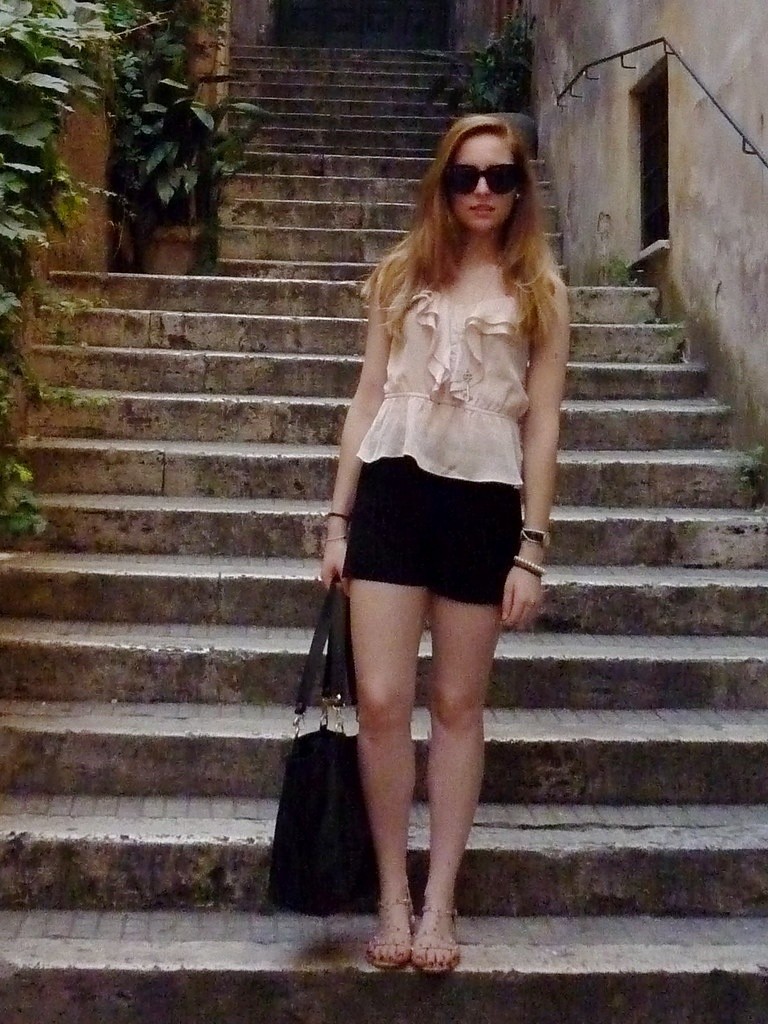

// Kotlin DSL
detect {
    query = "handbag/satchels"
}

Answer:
[266,580,380,917]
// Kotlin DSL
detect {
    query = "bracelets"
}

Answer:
[514,562,542,577]
[327,513,348,521]
[514,556,547,575]
[326,536,346,542]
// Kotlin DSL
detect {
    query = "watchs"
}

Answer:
[521,529,551,548]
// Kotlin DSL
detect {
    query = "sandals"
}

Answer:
[364,887,416,970]
[411,906,461,973]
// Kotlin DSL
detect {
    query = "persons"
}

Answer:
[321,117,570,972]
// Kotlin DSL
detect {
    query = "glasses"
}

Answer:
[441,164,522,194]
